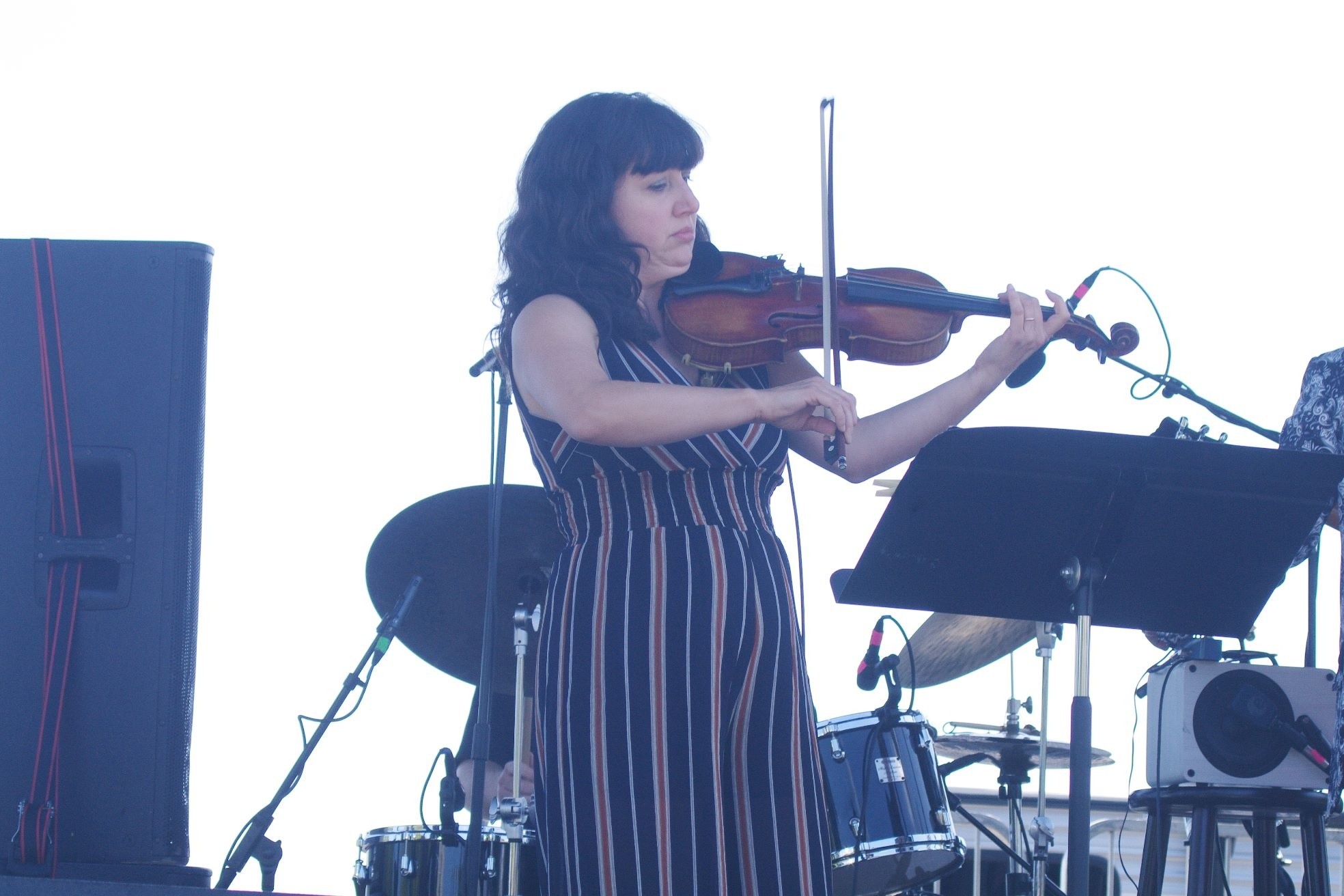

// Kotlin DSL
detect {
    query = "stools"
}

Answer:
[1128,789,1343,896]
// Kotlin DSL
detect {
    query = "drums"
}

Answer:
[814,710,968,896]
[352,824,538,896]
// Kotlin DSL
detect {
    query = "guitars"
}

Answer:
[1149,416,1340,531]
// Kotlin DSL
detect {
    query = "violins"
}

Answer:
[657,240,1141,380]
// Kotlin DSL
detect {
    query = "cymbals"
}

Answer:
[933,734,1116,769]
[363,486,572,700]
[892,612,1062,689]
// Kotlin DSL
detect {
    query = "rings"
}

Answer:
[1024,317,1035,321]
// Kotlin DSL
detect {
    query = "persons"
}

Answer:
[1279,348,1344,829]
[454,685,535,821]
[489,93,1071,896]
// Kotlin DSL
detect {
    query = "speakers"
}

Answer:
[0,236,215,867]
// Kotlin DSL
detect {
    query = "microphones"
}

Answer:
[1004,269,1101,388]
[441,749,466,813]
[1228,683,1331,774]
[856,616,886,691]
